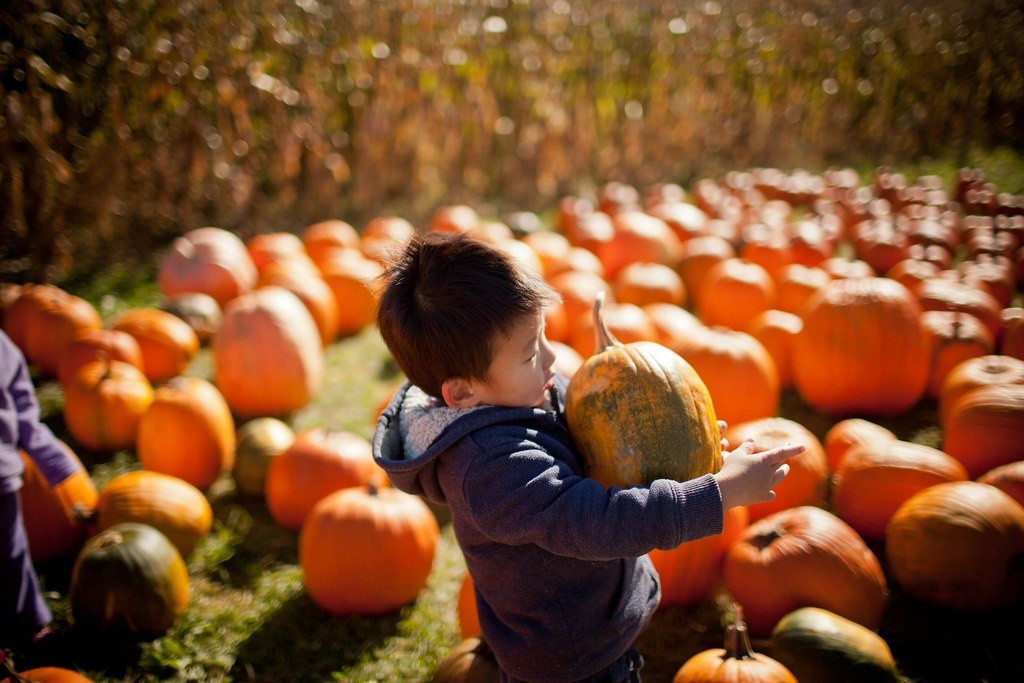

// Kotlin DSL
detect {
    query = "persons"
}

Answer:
[374,230,808,683]
[1,326,101,672]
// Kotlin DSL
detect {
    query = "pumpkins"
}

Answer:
[0,202,501,683]
[484,165,1024,683]
